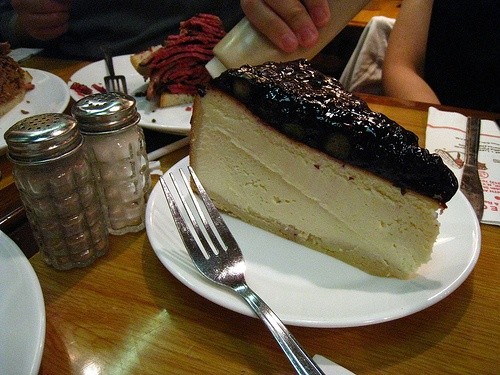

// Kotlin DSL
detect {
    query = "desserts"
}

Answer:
[189,58,459,279]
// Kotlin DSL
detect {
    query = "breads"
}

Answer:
[0,42,35,118]
[130,44,196,108]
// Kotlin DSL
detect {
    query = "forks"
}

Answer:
[159,165,325,375]
[101,43,128,95]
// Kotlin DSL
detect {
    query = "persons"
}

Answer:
[383,0,500,112]
[0,0,331,62]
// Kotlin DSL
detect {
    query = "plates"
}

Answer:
[67,54,192,136]
[0,67,71,155]
[0,230,46,375]
[144,156,483,330]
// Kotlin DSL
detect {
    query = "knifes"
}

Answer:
[460,115,484,221]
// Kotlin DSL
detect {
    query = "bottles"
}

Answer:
[203,0,371,79]
[3,113,110,271]
[71,92,152,234]
